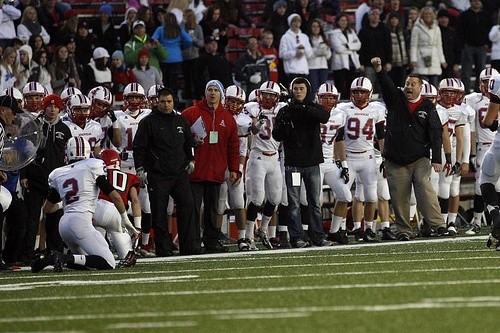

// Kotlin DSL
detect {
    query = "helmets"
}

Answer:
[0,67,500,123]
[64,136,90,160]
[100,150,121,167]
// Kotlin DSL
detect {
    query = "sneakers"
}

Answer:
[0,227,500,274]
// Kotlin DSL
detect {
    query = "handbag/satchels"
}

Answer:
[423,56,431,67]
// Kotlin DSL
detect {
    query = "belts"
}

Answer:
[475,143,491,146]
[263,152,276,156]
[350,150,368,154]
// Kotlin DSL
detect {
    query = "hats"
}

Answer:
[77,22,88,31]
[18,44,32,72]
[63,10,77,20]
[204,36,216,43]
[61,34,76,46]
[273,0,287,10]
[132,21,144,29]
[93,48,150,60]
[100,5,111,17]
[436,9,448,19]
[287,14,301,24]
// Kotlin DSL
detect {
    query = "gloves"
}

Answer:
[229,170,242,189]
[258,115,268,125]
[289,104,306,111]
[334,158,460,185]
[282,109,288,120]
[120,211,139,236]
[186,161,195,175]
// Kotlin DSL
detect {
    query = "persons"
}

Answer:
[0,0,234,113]
[0,80,289,271]
[231,0,500,101]
[272,67,500,250]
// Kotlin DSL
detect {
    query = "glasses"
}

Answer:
[296,35,299,44]
